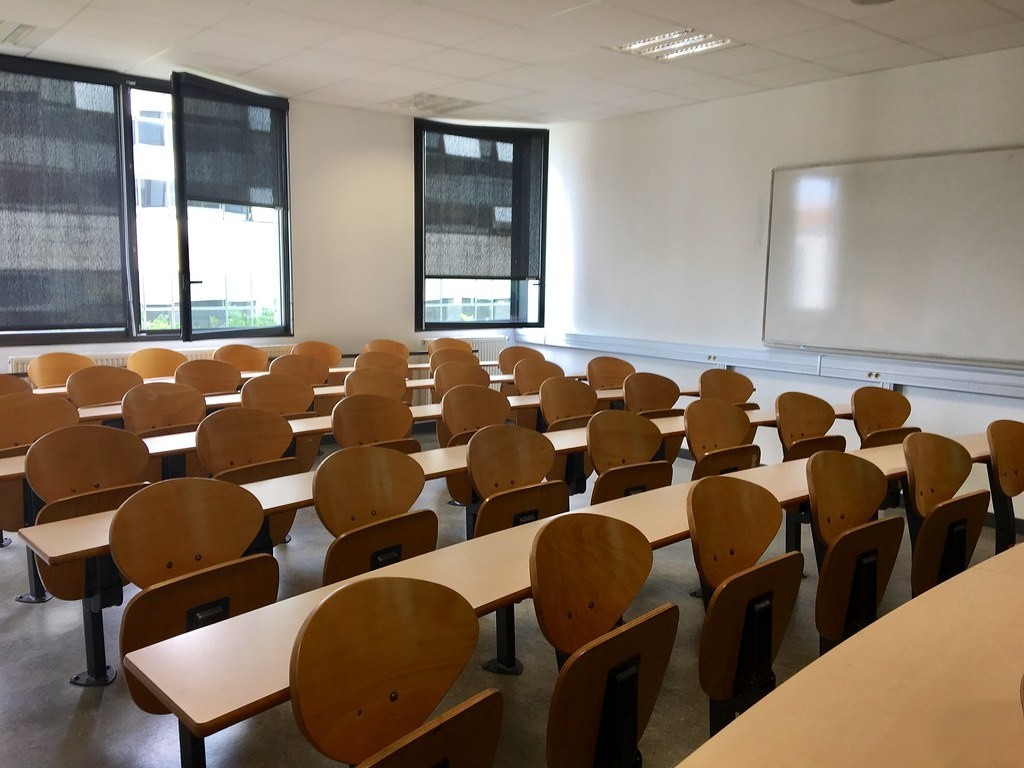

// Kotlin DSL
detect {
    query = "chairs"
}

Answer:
[285,573,502,767]
[1,338,922,723]
[806,448,905,657]
[529,511,679,768]
[686,476,805,738]
[903,433,991,598]
[986,420,1024,554]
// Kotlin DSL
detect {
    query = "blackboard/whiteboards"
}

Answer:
[761,143,1024,371]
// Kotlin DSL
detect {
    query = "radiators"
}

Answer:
[419,336,511,405]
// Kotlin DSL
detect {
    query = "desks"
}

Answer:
[0,360,1024,768]
